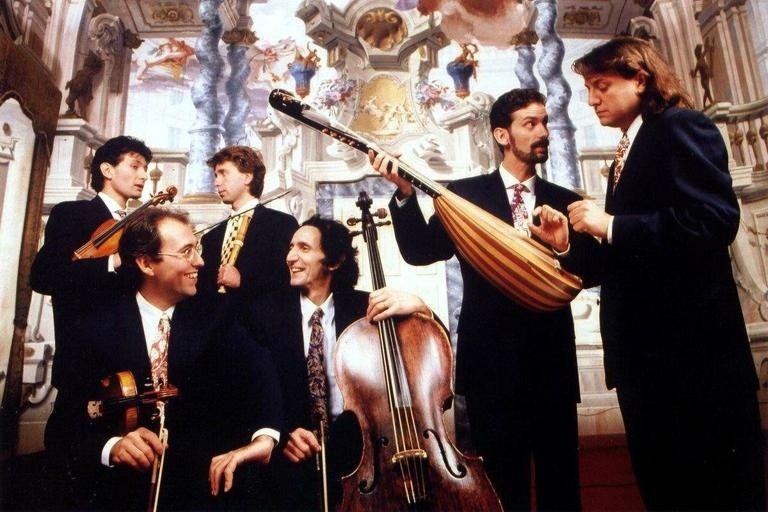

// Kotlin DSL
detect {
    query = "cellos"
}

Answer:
[332,192,504,511]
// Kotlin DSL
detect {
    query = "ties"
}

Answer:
[613,135,629,193]
[115,210,127,219]
[305,307,330,450]
[220,215,240,267]
[511,184,531,238]
[150,314,171,413]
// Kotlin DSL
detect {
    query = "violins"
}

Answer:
[86,371,180,437]
[71,186,177,262]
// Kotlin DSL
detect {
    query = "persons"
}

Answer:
[363,86,607,510]
[565,36,767,510]
[259,210,457,511]
[27,134,155,389]
[41,207,284,512]
[689,37,716,112]
[193,143,303,297]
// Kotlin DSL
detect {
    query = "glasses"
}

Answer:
[157,244,203,261]
